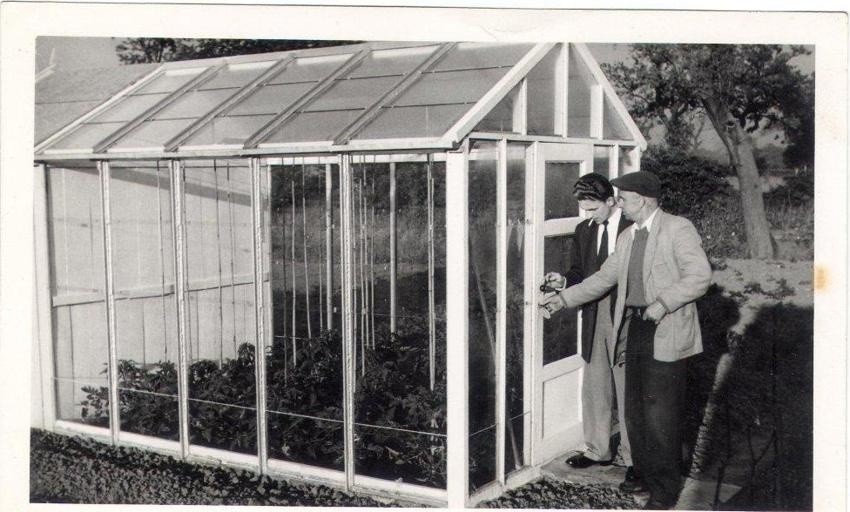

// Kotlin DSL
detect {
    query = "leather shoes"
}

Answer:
[618,478,648,492]
[643,494,669,509]
[565,453,614,468]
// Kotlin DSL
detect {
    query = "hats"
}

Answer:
[610,170,663,199]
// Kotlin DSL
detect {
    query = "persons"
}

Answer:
[539,170,715,510]
[541,171,653,469]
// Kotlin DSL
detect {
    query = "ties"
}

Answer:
[597,219,608,269]
